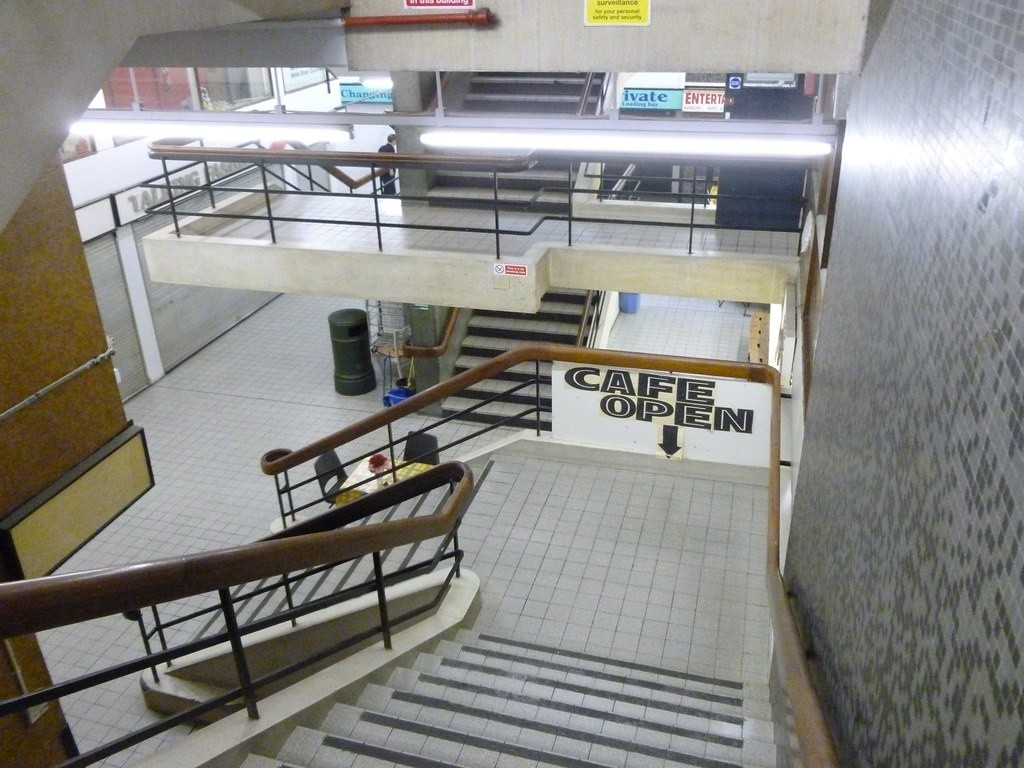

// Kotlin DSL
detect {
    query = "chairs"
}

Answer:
[402,431,440,466]
[313,449,349,509]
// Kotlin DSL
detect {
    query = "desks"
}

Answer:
[748,313,769,384]
[376,342,409,400]
[335,454,435,509]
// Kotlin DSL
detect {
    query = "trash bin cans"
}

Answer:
[619,292,641,314]
[328,308,377,396]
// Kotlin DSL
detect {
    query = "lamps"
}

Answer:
[68,119,357,142]
[418,110,837,159]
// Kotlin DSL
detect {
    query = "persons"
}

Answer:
[379,134,396,195]
